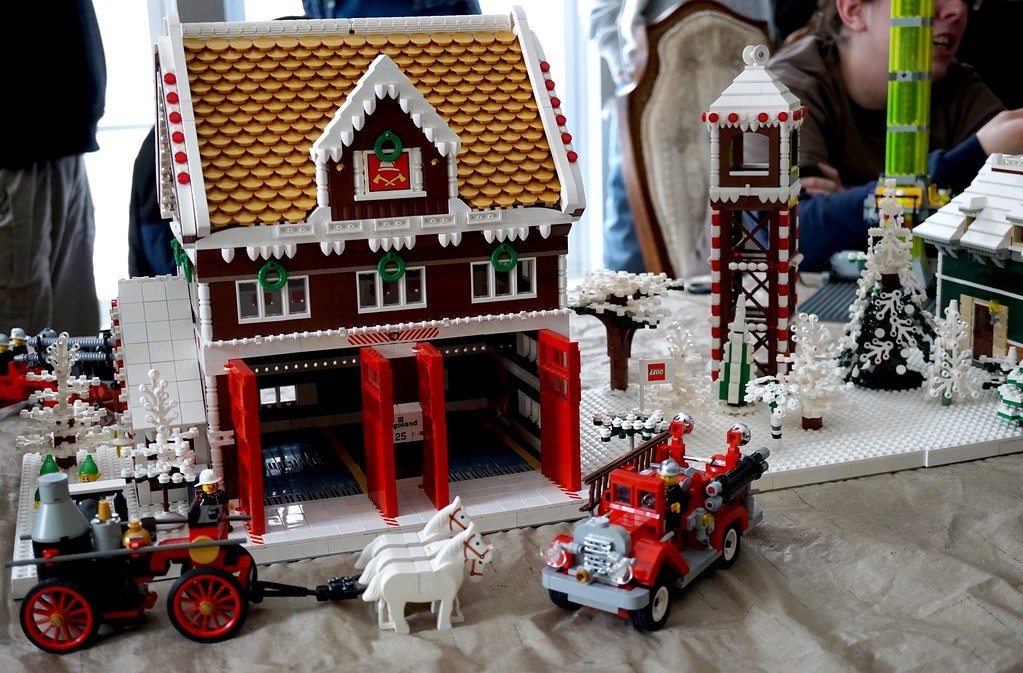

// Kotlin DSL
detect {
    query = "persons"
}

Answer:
[194,469,233,533]
[685,0,1023,283]
[586,1,778,271]
[642,459,688,538]
[0,0,107,338]
[0,328,31,376]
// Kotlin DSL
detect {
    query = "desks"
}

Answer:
[0,408,1023,673]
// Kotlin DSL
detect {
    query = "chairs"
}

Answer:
[613,0,773,277]
